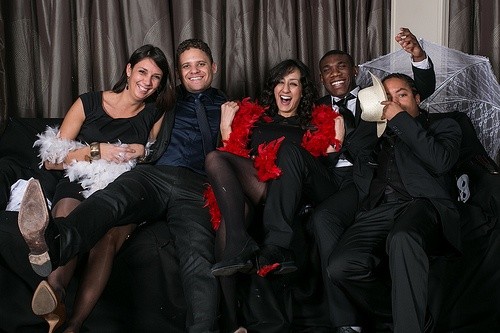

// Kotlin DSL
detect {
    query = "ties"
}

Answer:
[333,94,356,139]
[192,98,214,158]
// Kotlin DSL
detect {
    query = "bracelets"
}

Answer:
[90,142,101,160]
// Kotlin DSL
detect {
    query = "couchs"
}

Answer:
[0,111,500,333]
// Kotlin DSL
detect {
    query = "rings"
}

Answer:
[117,155,121,160]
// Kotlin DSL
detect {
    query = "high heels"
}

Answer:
[212,237,259,274]
[31,281,65,333]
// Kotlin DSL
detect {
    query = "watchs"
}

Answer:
[330,142,342,151]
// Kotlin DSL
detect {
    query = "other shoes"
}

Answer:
[18,178,59,277]
[227,238,298,273]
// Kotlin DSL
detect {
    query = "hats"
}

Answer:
[358,69,388,139]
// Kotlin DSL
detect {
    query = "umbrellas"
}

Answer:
[356,36,500,168]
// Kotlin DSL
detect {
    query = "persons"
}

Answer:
[32,45,174,333]
[241,28,437,317]
[202,59,345,333]
[17,38,248,333]
[325,72,462,333]
